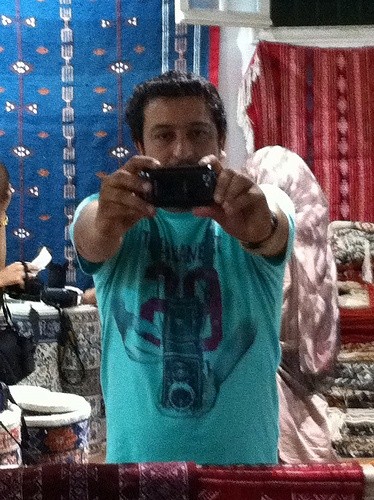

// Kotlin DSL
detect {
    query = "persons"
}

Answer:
[1,161,38,291]
[69,68,295,463]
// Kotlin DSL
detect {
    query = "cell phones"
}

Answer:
[141,164,215,206]
